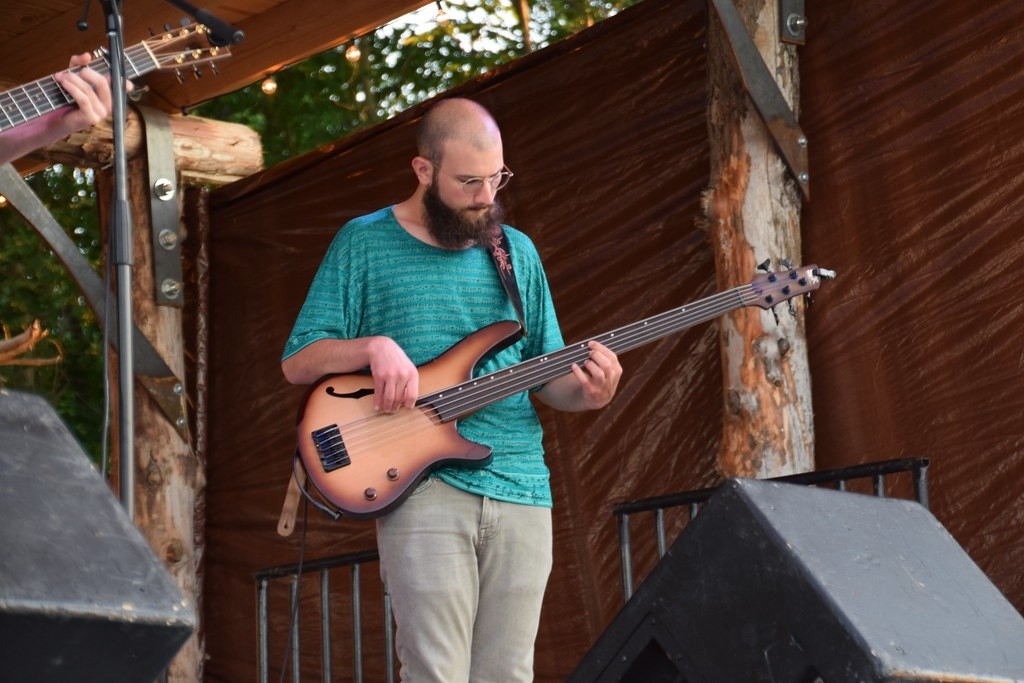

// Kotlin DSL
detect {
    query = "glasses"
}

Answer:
[427,164,513,196]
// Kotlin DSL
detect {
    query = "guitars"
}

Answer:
[0,15,232,133]
[294,258,837,519]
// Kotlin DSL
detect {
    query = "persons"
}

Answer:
[0,52,133,165]
[282,98,622,683]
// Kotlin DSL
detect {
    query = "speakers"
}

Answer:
[0,388,196,683]
[563,477,1024,683]
